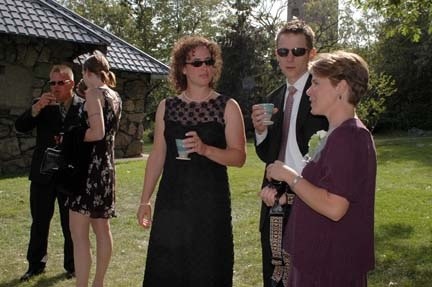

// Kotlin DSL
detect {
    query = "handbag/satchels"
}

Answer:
[39,134,76,178]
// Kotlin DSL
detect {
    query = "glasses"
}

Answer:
[47,80,72,86]
[185,59,215,67]
[277,47,312,58]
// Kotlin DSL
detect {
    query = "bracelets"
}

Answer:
[140,202,151,206]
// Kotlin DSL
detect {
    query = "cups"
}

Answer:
[76,79,87,96]
[175,138,193,161]
[258,103,275,126]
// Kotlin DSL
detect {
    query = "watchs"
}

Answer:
[290,176,303,190]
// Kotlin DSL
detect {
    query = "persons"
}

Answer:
[136,34,247,287]
[251,19,329,287]
[265,51,377,287]
[14,63,86,281]
[54,55,123,287]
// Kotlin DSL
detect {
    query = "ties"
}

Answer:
[278,86,297,163]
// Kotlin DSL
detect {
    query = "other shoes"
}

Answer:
[67,271,74,279]
[20,268,46,281]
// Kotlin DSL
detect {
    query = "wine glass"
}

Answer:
[267,179,286,215]
